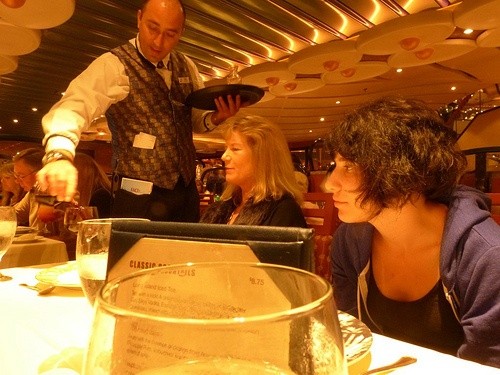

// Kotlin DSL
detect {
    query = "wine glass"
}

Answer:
[63,206,99,255]
[0,206,17,281]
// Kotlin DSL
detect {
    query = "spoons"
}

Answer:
[19,282,56,295]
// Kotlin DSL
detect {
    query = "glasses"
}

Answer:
[13,168,39,184]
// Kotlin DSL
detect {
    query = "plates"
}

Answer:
[336,309,373,366]
[186,84,265,111]
[35,262,81,289]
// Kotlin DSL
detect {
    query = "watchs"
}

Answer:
[42,149,74,166]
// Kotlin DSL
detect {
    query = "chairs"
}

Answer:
[298,191,337,287]
[198,192,216,221]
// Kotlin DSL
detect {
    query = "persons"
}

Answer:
[324,98,500,370]
[0,148,112,260]
[203,115,316,306]
[36,0,250,223]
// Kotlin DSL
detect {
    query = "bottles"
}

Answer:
[225,65,242,84]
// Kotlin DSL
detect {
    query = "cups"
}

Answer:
[82,261,348,375]
[76,218,151,308]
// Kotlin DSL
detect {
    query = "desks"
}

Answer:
[0,224,500,375]
